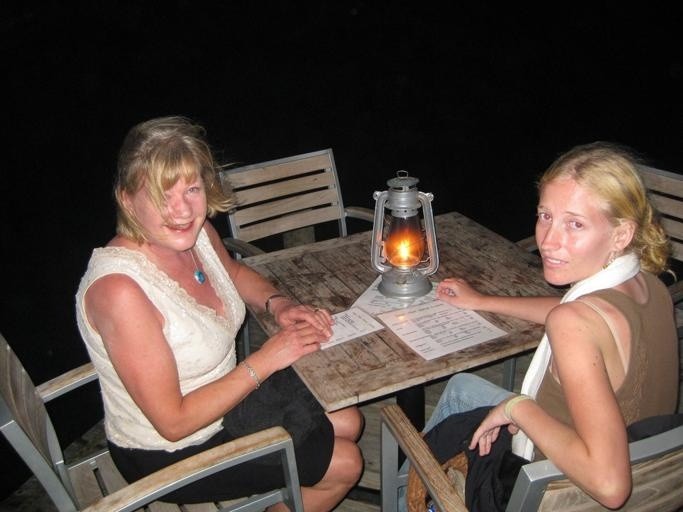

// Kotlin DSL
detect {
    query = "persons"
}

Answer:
[399,142,680,512]
[73,116,366,512]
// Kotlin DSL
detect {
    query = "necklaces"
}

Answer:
[153,244,209,287]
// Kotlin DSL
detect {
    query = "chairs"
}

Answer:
[220,149,385,352]
[2,336,303,511]
[379,403,683,512]
[497,164,683,388]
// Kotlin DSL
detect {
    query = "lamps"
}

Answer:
[369,170,440,301]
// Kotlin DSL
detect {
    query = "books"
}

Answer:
[313,272,508,361]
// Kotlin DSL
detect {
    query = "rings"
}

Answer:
[311,308,320,314]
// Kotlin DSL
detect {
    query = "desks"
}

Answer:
[230,210,570,512]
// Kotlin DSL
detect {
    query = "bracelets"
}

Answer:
[262,293,290,314]
[238,360,264,392]
[503,394,533,425]
